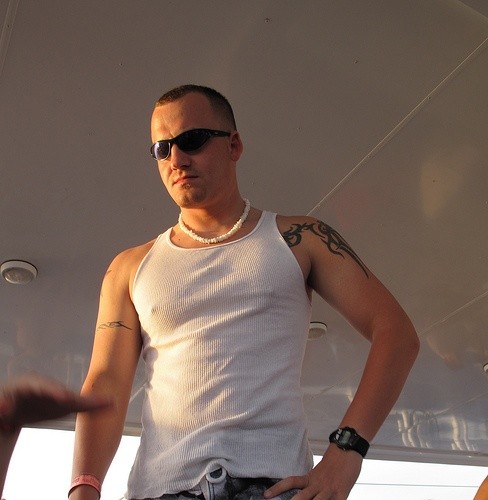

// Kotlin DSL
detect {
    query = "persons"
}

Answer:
[68,85,420,500]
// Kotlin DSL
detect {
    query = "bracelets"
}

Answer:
[68,474,101,500]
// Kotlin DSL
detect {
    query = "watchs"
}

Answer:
[329,426,370,458]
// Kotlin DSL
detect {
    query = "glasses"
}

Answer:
[150,128,230,161]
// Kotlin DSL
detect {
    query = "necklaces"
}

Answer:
[179,198,251,244]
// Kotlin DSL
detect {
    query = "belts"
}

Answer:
[177,470,266,499]
